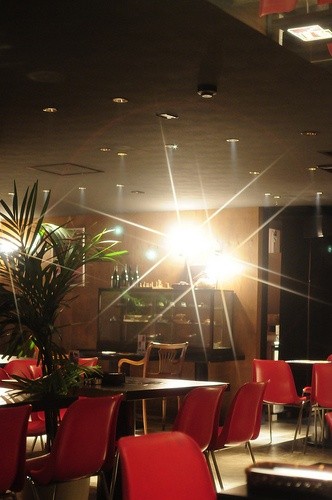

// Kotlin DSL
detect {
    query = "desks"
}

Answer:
[92,349,161,376]
[283,359,332,448]
[1,376,230,500]
[0,386,80,452]
[79,346,246,381]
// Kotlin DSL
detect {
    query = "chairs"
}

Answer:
[0,342,332,500]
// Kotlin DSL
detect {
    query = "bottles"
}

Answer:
[112,263,140,288]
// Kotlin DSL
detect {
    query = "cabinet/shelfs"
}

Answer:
[96,286,235,354]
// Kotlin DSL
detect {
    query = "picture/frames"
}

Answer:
[49,226,86,287]
